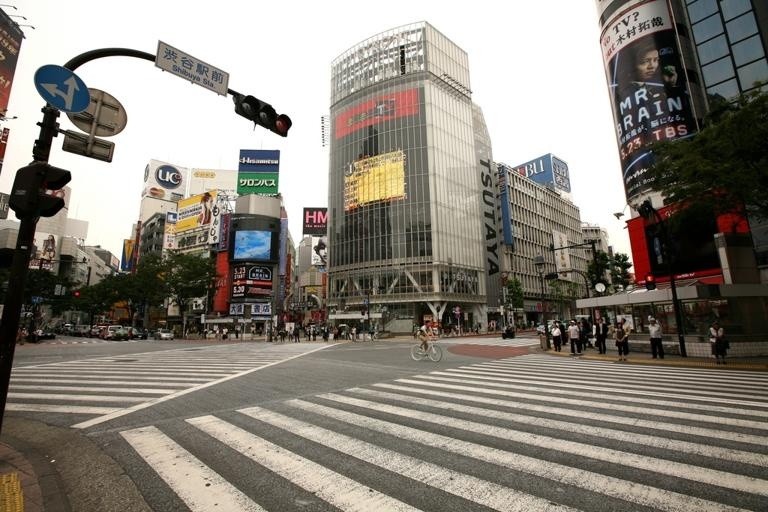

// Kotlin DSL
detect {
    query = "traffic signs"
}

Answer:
[31,296,45,304]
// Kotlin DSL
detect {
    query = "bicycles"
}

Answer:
[410,336,442,362]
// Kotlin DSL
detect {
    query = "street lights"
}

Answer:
[533,249,548,325]
[365,286,377,319]
[544,269,594,327]
[31,235,55,271]
[637,201,687,357]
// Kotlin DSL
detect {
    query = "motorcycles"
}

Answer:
[501,326,516,339]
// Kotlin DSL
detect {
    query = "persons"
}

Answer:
[616,40,692,145]
[414,318,545,335]
[29,238,37,261]
[548,315,632,361]
[177,321,376,342]
[707,319,727,365]
[196,192,213,227]
[648,318,665,359]
[417,320,436,358]
[313,238,328,265]
[38,235,56,271]
[17,322,147,345]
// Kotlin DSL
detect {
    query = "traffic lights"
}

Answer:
[73,291,81,298]
[10,161,72,224]
[642,272,658,291]
[232,93,292,137]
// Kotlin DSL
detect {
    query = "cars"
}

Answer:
[150,326,175,340]
[32,319,148,342]
[536,314,608,339]
